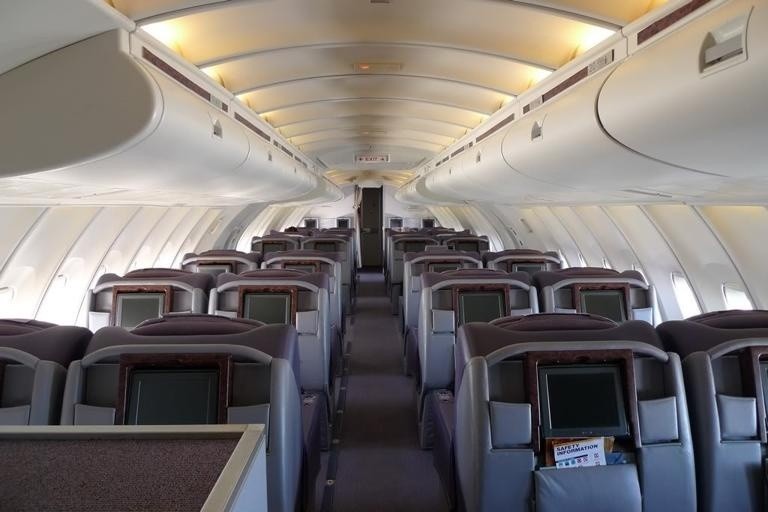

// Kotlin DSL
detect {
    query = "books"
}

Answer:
[543,435,627,469]
